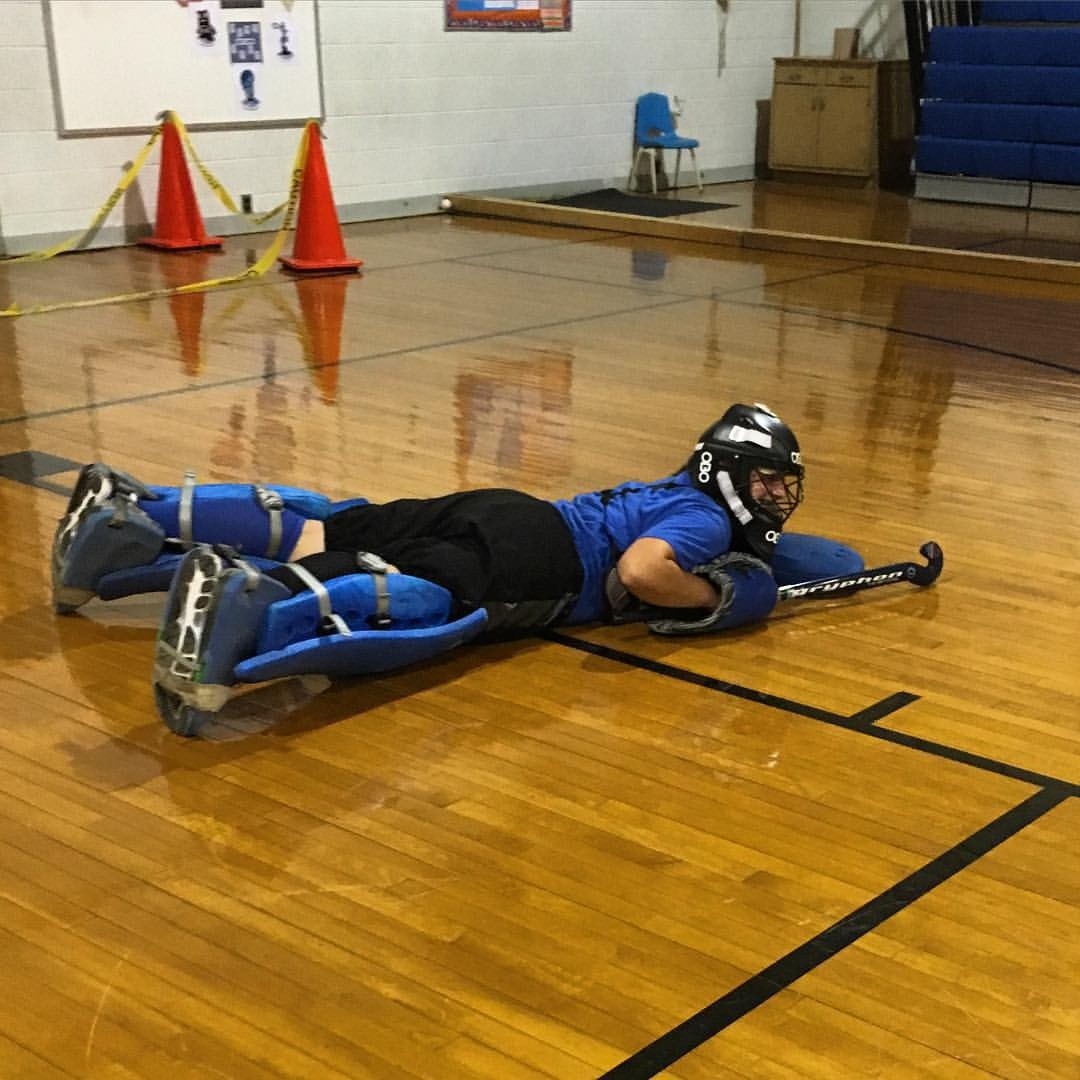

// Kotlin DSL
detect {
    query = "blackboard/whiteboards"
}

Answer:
[41,0,325,140]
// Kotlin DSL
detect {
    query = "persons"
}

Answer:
[47,404,868,738]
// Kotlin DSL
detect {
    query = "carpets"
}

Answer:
[536,187,742,218]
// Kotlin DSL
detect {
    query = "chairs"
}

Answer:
[627,92,705,198]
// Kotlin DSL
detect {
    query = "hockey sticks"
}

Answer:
[609,539,946,626]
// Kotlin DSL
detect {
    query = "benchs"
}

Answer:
[912,0,1080,214]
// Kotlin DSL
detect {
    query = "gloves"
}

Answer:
[762,530,866,581]
[649,555,776,636]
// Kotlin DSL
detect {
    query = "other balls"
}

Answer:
[441,198,452,209]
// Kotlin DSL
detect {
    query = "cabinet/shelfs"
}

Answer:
[766,54,884,192]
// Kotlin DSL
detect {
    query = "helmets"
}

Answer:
[691,403,806,565]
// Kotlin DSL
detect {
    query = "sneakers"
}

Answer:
[49,470,139,615]
[155,553,230,737]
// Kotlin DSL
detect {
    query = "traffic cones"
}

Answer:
[276,124,363,271]
[135,111,225,250]
